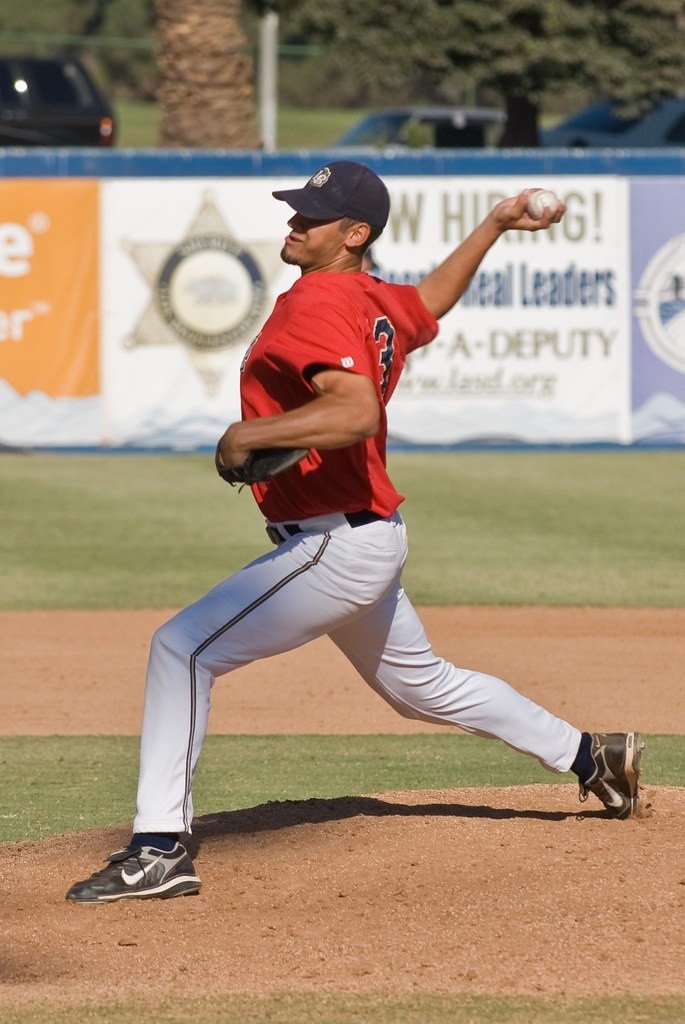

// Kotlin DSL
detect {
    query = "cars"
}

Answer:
[1,53,118,148]
[328,107,505,146]
[545,94,685,148]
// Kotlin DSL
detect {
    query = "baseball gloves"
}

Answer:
[214,435,311,484]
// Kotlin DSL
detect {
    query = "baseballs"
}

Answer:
[527,187,560,220]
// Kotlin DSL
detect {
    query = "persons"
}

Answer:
[66,161,642,904]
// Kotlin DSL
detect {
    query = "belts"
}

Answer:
[267,508,390,547]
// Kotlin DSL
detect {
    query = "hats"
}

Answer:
[273,159,390,233]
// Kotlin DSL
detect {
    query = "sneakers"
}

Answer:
[578,731,648,819]
[67,839,203,902]
